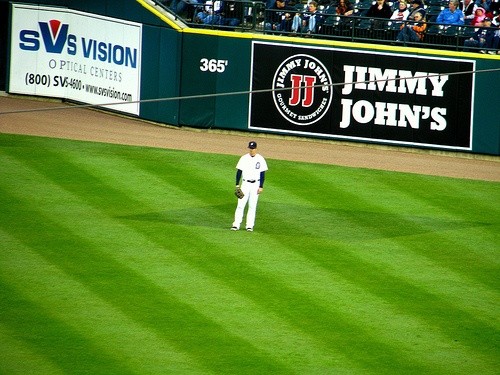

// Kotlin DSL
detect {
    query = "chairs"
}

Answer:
[244,0,475,50]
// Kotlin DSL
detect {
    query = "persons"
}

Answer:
[151,0,500,55]
[230,141,269,232]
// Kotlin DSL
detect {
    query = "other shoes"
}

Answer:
[247,228,254,232]
[231,226,239,231]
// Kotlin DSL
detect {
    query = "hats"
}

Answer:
[248,141,257,149]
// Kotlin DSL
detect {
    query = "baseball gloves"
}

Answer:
[234,187,245,199]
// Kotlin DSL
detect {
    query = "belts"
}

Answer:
[242,179,260,183]
